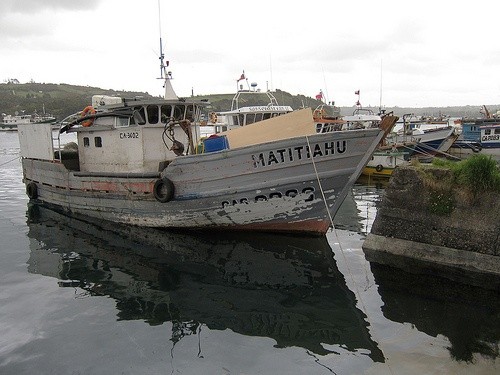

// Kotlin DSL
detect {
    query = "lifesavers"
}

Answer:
[153,178,174,203]
[26,183,38,198]
[80,106,95,126]
[376,164,384,171]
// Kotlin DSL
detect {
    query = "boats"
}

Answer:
[0,113,60,130]
[28,202,386,365]
[17,36,398,237]
[198,69,500,178]
